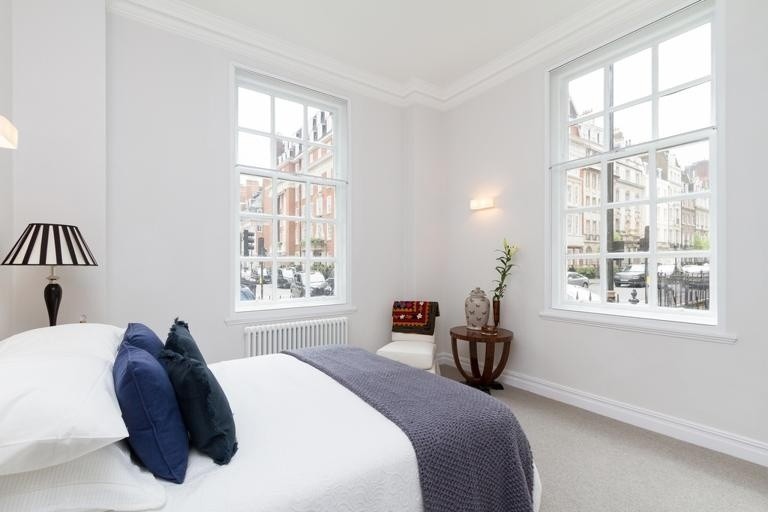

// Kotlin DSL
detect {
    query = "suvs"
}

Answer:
[277,268,294,289]
[252,268,272,284]
[290,270,333,298]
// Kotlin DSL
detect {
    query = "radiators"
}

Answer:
[244,316,349,357]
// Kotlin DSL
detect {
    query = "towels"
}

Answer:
[392,301,440,335]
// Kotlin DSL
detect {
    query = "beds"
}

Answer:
[143,345,542,512]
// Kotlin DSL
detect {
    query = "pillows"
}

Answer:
[2,323,129,473]
[2,440,167,510]
[113,319,238,485]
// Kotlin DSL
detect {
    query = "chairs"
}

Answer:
[376,315,437,374]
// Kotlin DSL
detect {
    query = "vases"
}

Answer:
[493,300,500,328]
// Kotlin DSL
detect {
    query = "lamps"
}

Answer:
[470,199,494,210]
[1,223,98,326]
[0,115,17,149]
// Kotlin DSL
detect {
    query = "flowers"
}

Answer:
[491,238,520,300]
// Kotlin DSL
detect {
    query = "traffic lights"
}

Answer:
[244,229,255,256]
[258,238,268,256]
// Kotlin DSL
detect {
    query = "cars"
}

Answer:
[240,272,256,289]
[240,284,256,301]
[567,270,591,287]
[657,262,682,282]
[326,277,334,295]
[614,263,661,287]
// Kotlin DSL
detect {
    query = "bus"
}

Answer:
[681,256,709,288]
